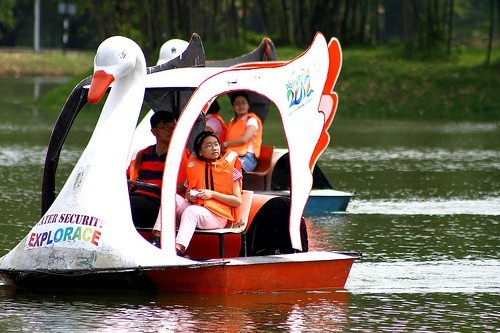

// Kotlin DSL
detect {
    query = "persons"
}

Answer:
[222,92,262,173]
[205,99,227,143]
[128,110,188,228]
[151,131,242,257]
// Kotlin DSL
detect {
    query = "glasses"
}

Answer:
[201,142,219,149]
[157,124,174,131]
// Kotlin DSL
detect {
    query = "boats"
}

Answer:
[0,32,362,297]
[133,37,354,216]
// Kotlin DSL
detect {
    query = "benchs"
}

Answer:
[136,190,254,258]
[242,145,275,191]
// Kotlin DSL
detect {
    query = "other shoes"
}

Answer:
[176,248,182,256]
[150,236,160,248]
